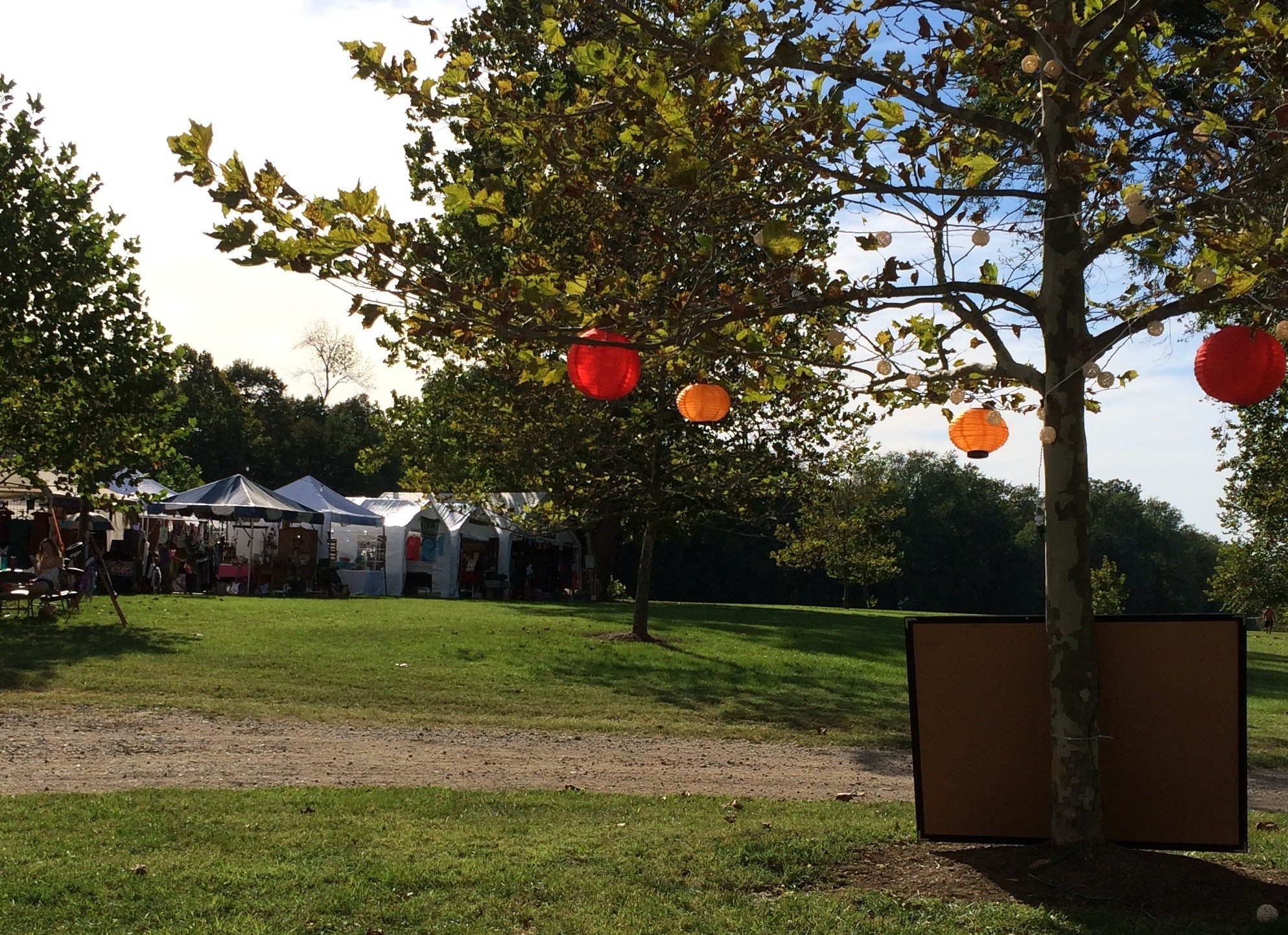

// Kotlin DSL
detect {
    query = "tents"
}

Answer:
[0,457,600,595]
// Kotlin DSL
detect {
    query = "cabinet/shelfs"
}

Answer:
[356,541,385,570]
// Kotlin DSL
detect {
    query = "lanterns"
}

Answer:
[568,328,639,398]
[676,383,730,421]
[1193,324,1286,406]
[949,404,1009,459]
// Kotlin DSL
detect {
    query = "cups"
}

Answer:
[11,556,16,570]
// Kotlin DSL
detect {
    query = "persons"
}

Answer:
[1,538,61,594]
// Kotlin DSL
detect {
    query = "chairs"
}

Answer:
[327,539,339,564]
[0,567,86,617]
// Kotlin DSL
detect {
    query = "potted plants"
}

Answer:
[483,573,508,588]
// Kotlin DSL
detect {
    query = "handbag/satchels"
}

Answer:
[154,519,209,553]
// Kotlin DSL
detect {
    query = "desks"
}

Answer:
[406,572,433,598]
[336,568,385,596]
[461,570,480,599]
[218,563,253,593]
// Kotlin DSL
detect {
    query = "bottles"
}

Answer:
[0,553,7,570]
